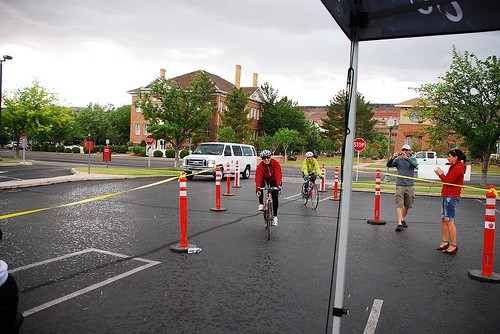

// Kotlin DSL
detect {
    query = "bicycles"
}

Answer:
[301,176,322,210]
[258,187,282,241]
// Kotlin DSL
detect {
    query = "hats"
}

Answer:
[401,145,411,150]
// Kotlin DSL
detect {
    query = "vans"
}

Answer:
[7,141,29,150]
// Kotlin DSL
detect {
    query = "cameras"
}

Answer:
[399,153,402,156]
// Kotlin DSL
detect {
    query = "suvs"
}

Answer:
[182,142,257,181]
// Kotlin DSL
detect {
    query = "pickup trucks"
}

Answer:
[411,151,464,170]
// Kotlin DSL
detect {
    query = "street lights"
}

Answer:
[384,118,395,182]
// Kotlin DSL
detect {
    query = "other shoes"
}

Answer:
[258,203,264,210]
[395,220,408,231]
[272,215,278,226]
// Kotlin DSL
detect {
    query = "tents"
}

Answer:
[320,0,496,334]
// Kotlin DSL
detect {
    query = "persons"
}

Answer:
[386,144,418,232]
[254,150,283,227]
[0,228,23,334]
[301,151,321,193]
[433,149,467,253]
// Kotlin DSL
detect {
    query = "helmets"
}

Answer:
[259,149,271,158]
[305,151,313,157]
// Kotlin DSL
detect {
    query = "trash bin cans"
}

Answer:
[103,146,111,161]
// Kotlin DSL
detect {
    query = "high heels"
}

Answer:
[436,239,458,255]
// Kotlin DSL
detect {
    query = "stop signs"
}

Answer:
[354,138,366,151]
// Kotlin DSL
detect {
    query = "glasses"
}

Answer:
[262,157,270,160]
